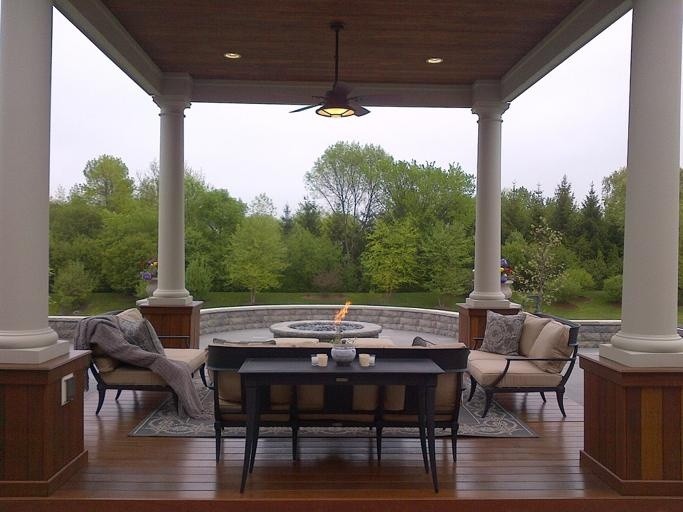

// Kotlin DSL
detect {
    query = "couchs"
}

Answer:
[466,312,581,418]
[207,337,470,464]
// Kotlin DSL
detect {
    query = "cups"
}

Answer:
[369,354,375,365]
[359,353,370,367]
[317,353,328,367]
[311,354,318,367]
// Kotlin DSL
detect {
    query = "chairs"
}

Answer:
[83,308,209,415]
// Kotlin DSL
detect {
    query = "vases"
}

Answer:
[146,278,157,297]
[502,281,513,299]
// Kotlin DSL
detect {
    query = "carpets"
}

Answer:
[127,376,541,437]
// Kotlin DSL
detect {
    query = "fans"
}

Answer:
[290,22,400,117]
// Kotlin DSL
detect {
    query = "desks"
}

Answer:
[237,358,448,493]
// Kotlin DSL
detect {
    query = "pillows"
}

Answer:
[119,317,168,358]
[529,320,570,373]
[514,310,551,357]
[411,337,435,346]
[211,338,278,346]
[477,309,526,356]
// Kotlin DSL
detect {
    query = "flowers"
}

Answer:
[140,257,158,280]
[500,259,511,282]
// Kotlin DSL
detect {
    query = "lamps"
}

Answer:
[314,93,355,117]
